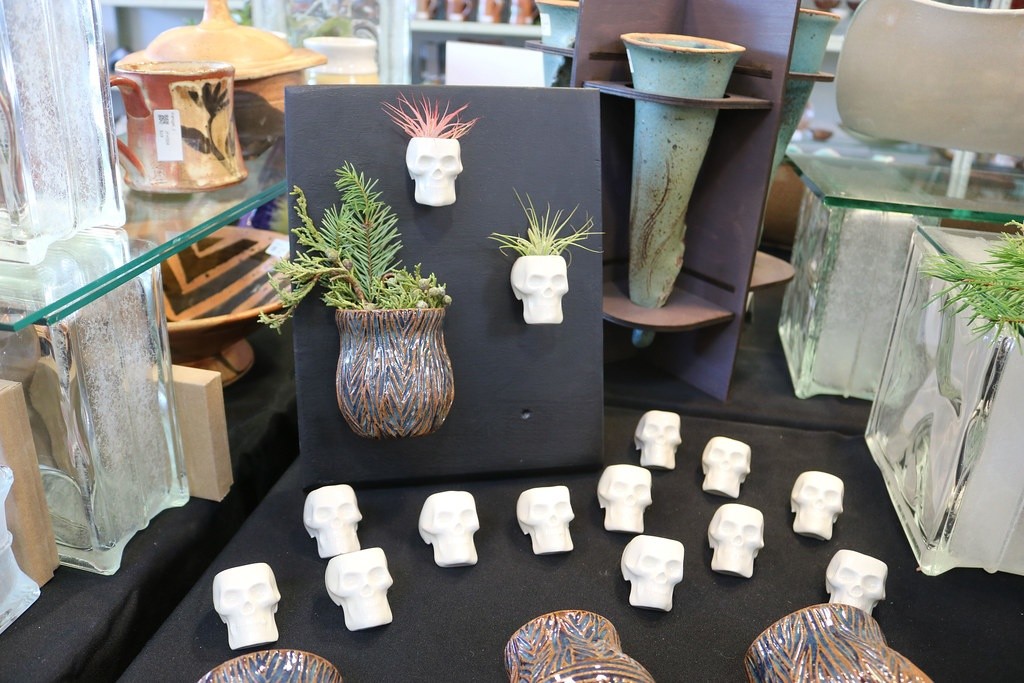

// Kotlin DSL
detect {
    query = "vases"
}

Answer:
[745,604,933,683]
[504,610,655,683]
[197,648,343,682]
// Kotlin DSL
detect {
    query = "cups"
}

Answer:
[510,0,538,23]
[444,0,472,22]
[478,0,506,23]
[413,0,438,18]
[305,38,378,85]
[110,61,247,194]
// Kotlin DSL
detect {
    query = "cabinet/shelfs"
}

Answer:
[568,1,801,404]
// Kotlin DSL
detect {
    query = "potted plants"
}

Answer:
[262,164,455,440]
[382,93,475,208]
[487,187,602,325]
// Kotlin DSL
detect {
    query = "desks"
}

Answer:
[0,285,1024,683]
